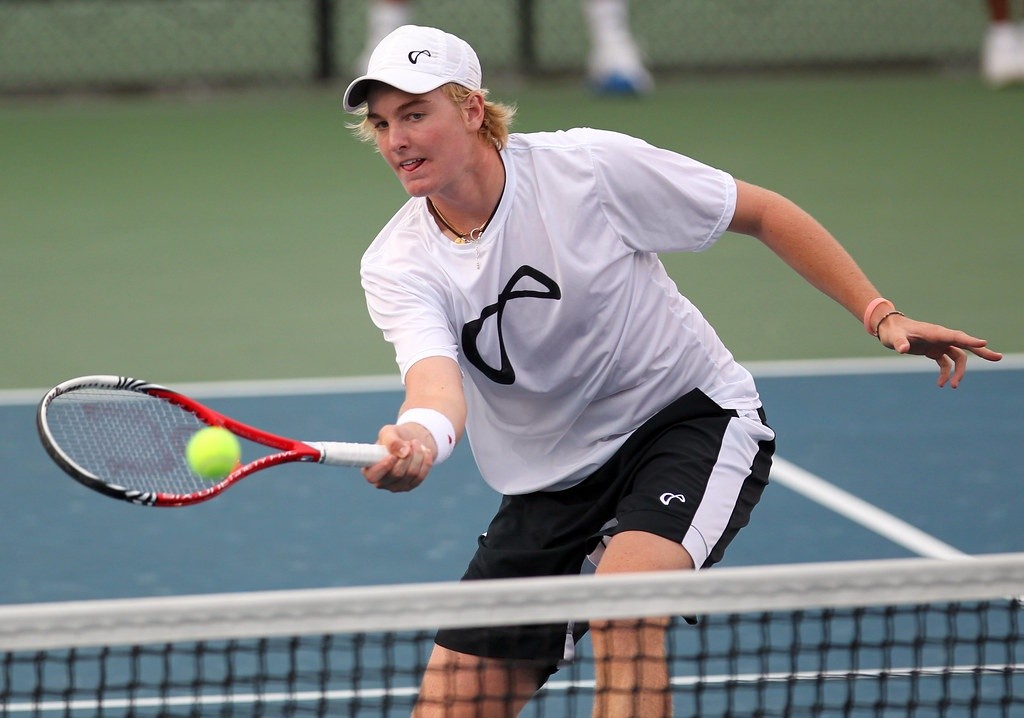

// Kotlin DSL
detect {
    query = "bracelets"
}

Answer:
[877,311,904,341]
[396,408,456,467]
[863,297,896,338]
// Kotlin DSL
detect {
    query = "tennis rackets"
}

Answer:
[36,375,391,509]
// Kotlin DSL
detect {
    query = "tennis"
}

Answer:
[183,426,241,478]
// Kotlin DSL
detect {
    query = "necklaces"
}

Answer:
[429,147,506,269]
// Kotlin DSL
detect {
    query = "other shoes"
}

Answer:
[977,19,1024,91]
[586,41,654,100]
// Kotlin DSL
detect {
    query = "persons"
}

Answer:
[982,0,1024,89]
[582,0,652,96]
[344,25,1001,718]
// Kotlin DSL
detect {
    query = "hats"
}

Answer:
[342,25,482,111]
